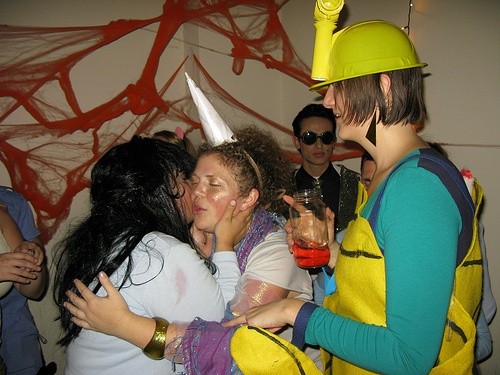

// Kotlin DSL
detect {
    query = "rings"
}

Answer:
[28,248,35,253]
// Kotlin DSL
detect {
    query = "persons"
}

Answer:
[50,134,259,375]
[281,103,498,375]
[151,126,197,160]
[220,20,486,375]
[0,184,57,375]
[62,124,324,375]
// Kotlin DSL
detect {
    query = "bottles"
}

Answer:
[288,189,330,268]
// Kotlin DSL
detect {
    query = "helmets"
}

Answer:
[309,0,427,91]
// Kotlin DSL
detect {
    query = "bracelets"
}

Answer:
[141,316,170,361]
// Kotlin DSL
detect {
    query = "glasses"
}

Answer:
[300,132,335,145]
